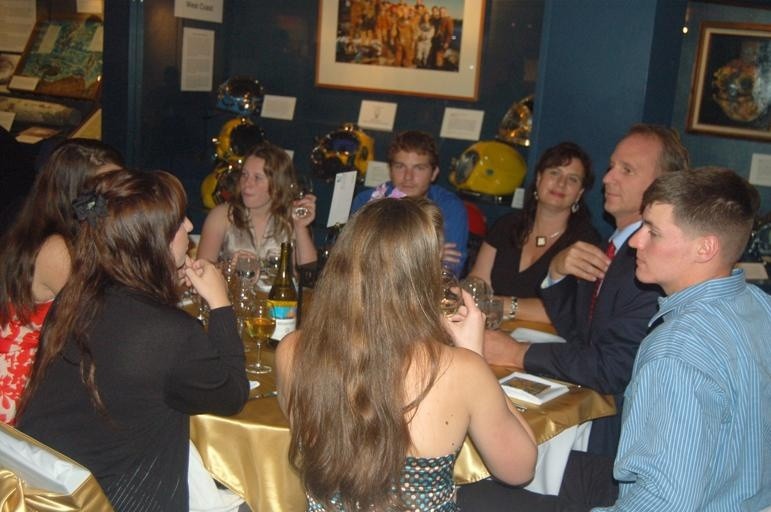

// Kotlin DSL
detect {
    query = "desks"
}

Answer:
[187,291,588,511]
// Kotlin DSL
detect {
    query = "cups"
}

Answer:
[439,269,505,330]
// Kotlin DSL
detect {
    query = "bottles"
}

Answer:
[267,243,300,348]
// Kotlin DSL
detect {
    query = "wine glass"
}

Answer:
[289,175,314,218]
[191,253,276,375]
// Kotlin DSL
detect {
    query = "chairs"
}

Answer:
[0,421,119,511]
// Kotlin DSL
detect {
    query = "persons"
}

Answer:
[470,143,607,325]
[274,193,538,512]
[344,129,469,287]
[588,164,771,512]
[2,144,126,428]
[192,143,320,290]
[482,121,694,396]
[346,0,454,68]
[12,167,248,512]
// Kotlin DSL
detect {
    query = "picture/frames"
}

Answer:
[316,0,487,103]
[687,18,770,141]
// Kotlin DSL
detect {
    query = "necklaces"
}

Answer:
[532,220,565,247]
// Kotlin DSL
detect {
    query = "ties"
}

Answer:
[588,239,616,319]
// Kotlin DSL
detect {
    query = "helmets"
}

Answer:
[212,117,265,163]
[494,94,535,147]
[310,122,376,185]
[448,140,528,195]
[201,161,243,210]
[215,74,264,116]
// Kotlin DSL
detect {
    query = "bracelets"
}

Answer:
[509,293,518,320]
